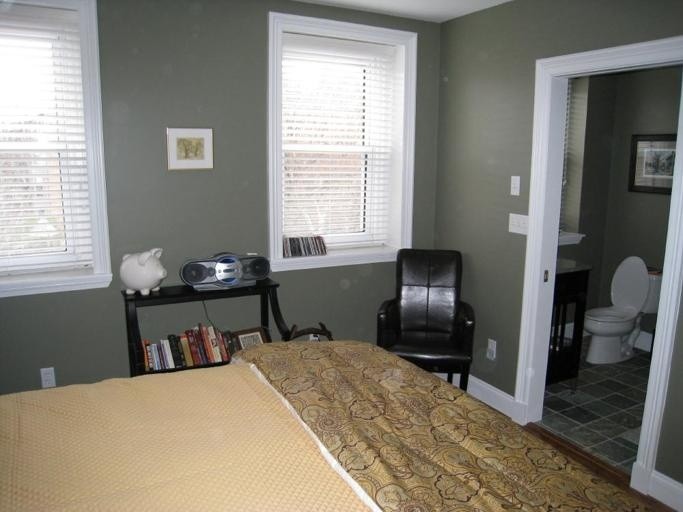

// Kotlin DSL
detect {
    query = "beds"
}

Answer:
[0,336,673,512]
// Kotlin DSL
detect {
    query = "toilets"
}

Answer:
[585,255,664,365]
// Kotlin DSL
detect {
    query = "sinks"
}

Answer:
[556,258,578,273]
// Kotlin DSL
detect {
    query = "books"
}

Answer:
[137,322,233,371]
[281,234,327,258]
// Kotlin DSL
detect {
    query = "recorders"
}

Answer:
[180,252,271,287]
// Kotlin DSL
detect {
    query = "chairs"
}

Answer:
[375,247,476,391]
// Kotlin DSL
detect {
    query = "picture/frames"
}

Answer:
[165,126,215,172]
[628,133,673,195]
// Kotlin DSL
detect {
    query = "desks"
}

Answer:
[120,276,281,377]
[546,255,593,370]
